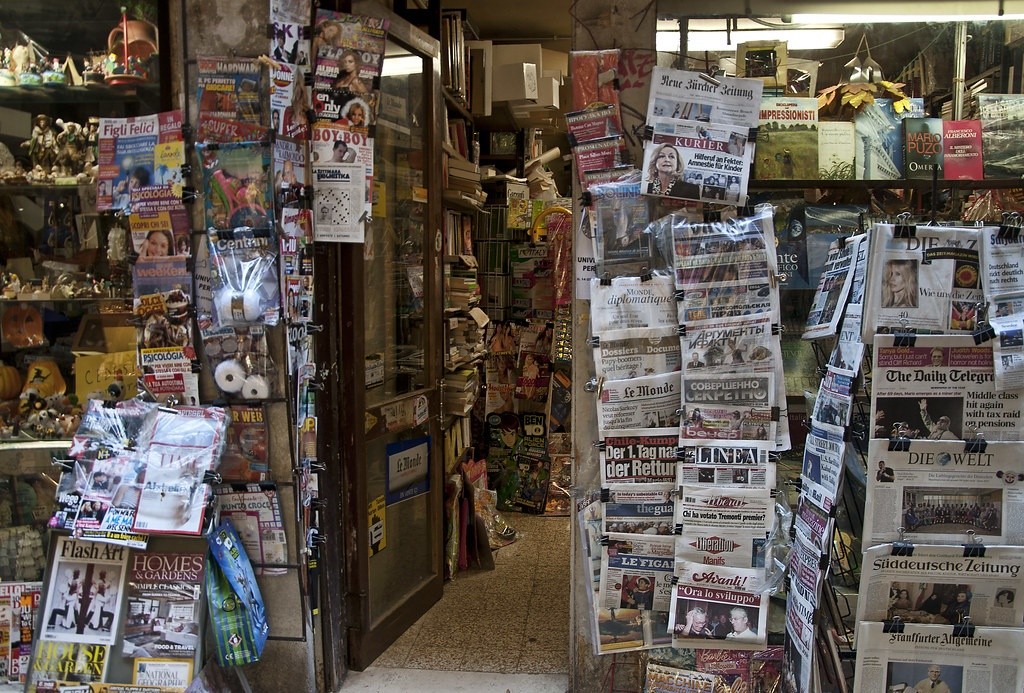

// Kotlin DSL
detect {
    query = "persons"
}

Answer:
[687,408,703,428]
[274,29,290,62]
[755,426,768,439]
[290,175,304,194]
[491,318,553,415]
[288,276,310,317]
[283,69,313,140]
[335,49,367,92]
[98,167,179,210]
[80,501,102,520]
[687,353,705,369]
[297,51,306,65]
[608,485,765,641]
[140,229,191,257]
[283,159,293,182]
[878,257,1018,693]
[19,113,101,173]
[336,97,371,128]
[720,340,747,365]
[725,410,745,430]
[311,19,342,68]
[646,142,740,202]
[272,109,280,132]
[328,140,357,163]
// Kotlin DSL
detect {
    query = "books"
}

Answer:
[269,9,389,242]
[47,398,226,547]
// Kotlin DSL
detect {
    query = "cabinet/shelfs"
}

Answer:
[1,0,188,451]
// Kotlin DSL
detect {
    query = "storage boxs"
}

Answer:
[754,98,818,131]
[490,64,538,103]
[510,77,560,115]
